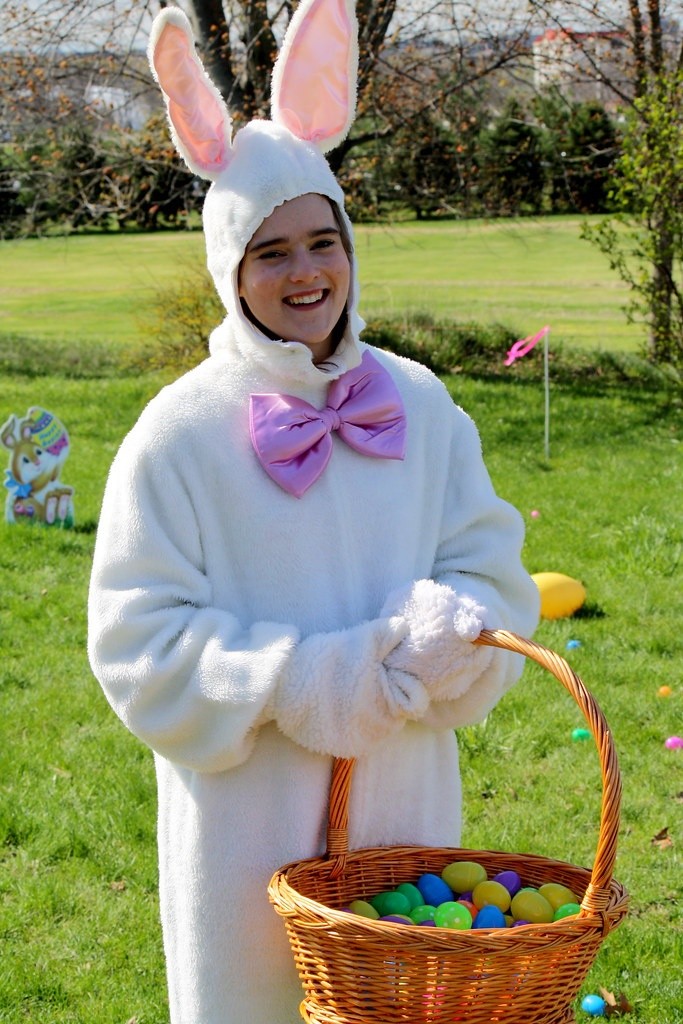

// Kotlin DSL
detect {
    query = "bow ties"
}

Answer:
[250,349,406,497]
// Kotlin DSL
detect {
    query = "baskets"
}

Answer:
[266,629,629,1024]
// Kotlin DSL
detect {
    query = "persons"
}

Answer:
[86,0,539,1024]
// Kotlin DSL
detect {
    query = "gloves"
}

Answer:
[269,616,429,759]
[380,580,494,702]
